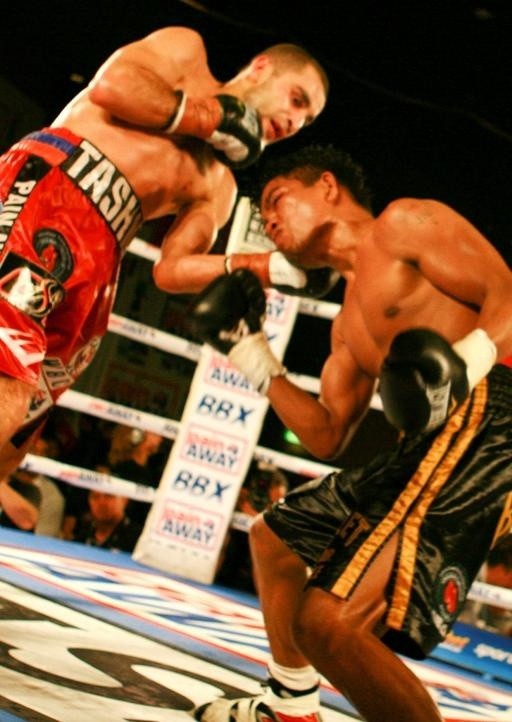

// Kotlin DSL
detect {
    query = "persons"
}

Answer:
[189,151,512,721]
[0,24,343,487]
[1,412,288,596]
[461,544,512,634]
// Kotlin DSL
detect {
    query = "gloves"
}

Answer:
[378,328,497,433]
[224,247,340,299]
[163,90,264,170]
[188,269,288,394]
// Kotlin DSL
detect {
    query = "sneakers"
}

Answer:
[192,675,324,722]
